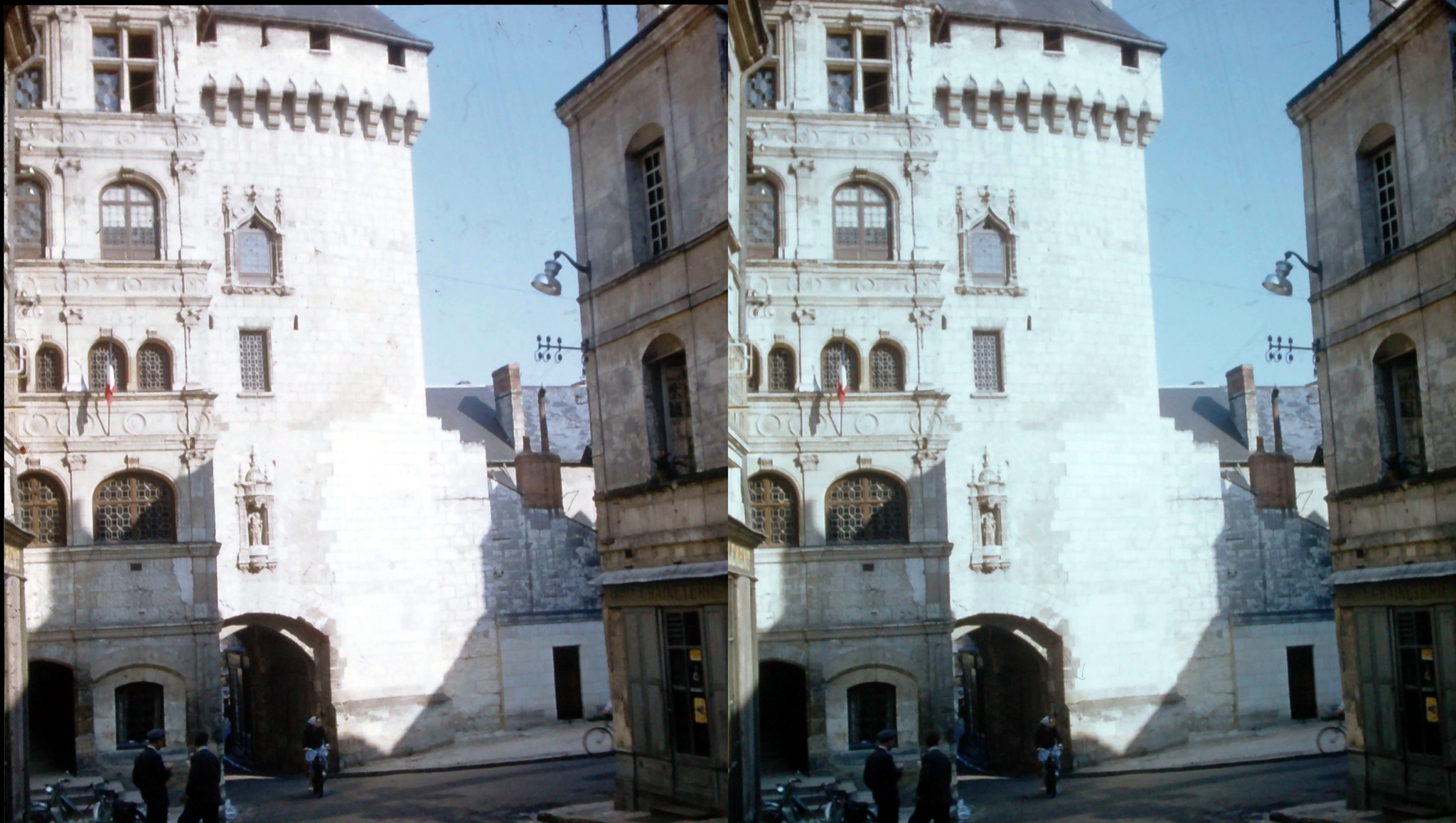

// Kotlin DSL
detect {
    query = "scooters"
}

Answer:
[759,772,878,823]
[27,774,146,823]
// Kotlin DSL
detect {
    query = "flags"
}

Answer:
[106,339,115,406]
[836,336,849,407]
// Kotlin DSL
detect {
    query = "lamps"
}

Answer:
[531,250,592,295]
[1262,251,1323,295]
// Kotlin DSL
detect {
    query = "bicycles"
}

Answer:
[1038,743,1063,798]
[583,712,615,755]
[1316,713,1348,755]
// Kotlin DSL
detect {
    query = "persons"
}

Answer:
[1334,700,1343,714]
[132,728,173,823]
[603,700,612,714]
[302,715,331,790]
[907,735,952,823]
[863,728,905,823]
[177,733,221,823]
[1033,715,1064,799]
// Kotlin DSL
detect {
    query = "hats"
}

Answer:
[877,729,897,742]
[147,728,165,742]
